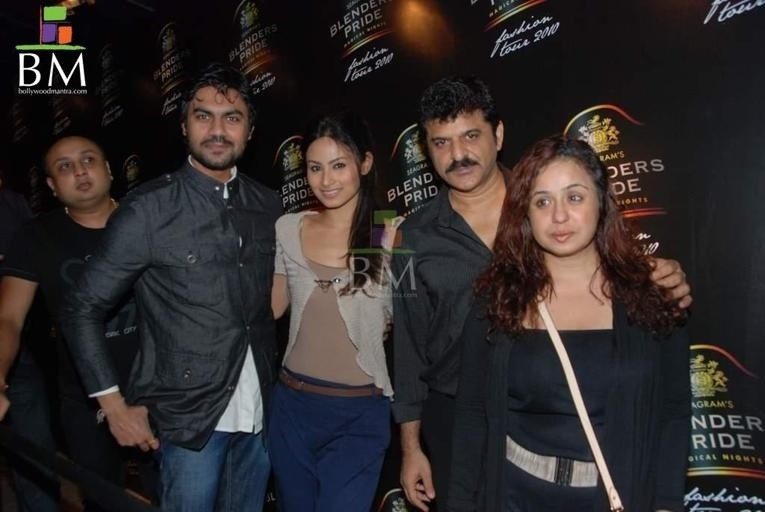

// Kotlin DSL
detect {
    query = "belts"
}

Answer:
[277,368,384,399]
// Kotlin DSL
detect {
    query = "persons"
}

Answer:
[443,136,694,510]
[386,75,695,510]
[269,118,412,511]
[55,66,277,512]
[0,130,152,510]
